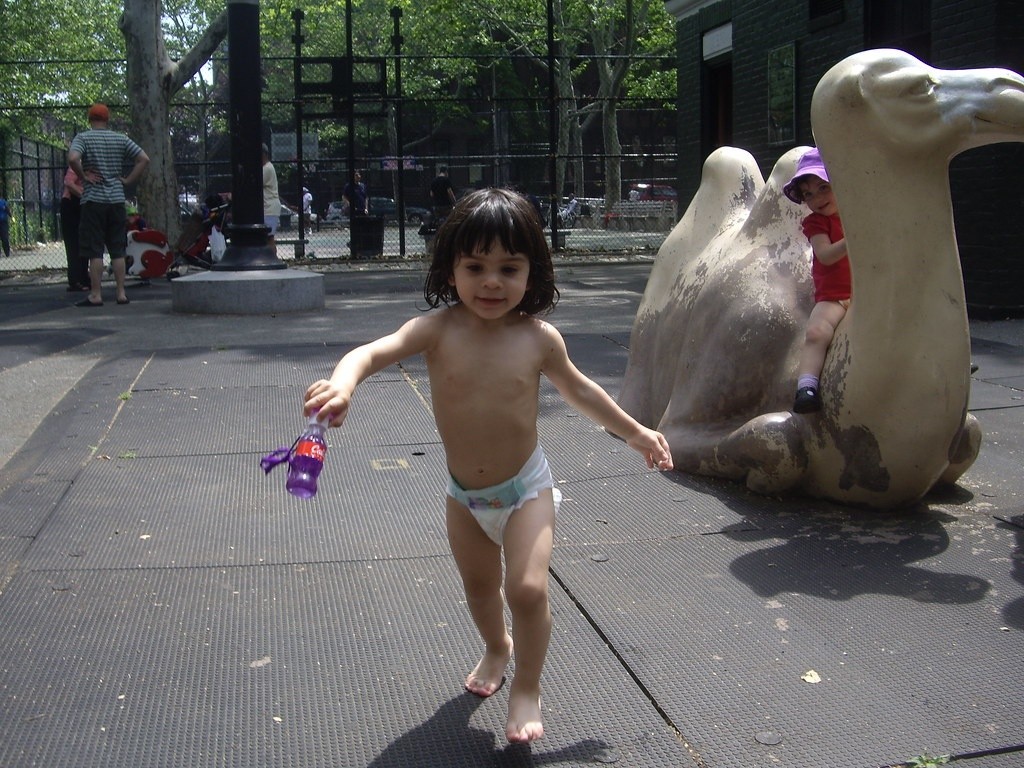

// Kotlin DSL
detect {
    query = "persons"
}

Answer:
[781,148,979,414]
[301,187,673,744]
[342,171,369,216]
[628,187,640,201]
[68,104,150,306]
[429,167,456,209]
[227,143,281,237]
[303,187,313,236]
[0,188,12,257]
[60,157,91,291]
[556,193,579,228]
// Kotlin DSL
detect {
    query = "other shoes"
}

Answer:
[970,363,979,374]
[793,386,823,414]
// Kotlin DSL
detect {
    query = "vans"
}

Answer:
[623,183,679,201]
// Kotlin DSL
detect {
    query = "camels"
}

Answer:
[604,47,1024,513]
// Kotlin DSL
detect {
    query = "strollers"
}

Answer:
[165,192,233,282]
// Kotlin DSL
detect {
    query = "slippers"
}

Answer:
[116,294,130,304]
[67,281,91,292]
[74,294,105,307]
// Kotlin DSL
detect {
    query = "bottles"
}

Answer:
[286,409,331,499]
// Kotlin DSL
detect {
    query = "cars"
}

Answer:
[368,197,430,225]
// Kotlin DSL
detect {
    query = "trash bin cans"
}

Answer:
[353,214,385,260]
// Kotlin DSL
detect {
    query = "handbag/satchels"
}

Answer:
[580,204,591,216]
[61,203,82,231]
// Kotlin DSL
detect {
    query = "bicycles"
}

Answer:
[545,201,576,229]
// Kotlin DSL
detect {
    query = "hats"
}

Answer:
[782,147,831,205]
[302,186,310,192]
[89,104,109,123]
